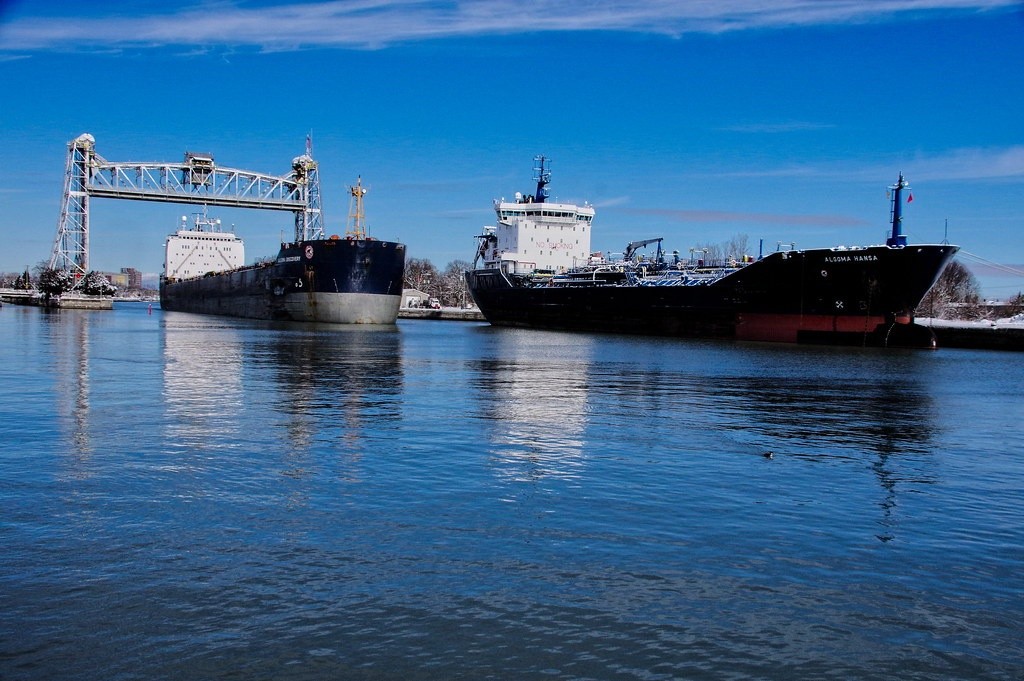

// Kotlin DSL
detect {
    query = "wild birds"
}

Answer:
[763,452,773,456]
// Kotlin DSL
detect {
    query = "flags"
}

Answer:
[907,192,914,202]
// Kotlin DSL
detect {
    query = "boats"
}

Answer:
[159,202,406,324]
[464,154,961,350]
